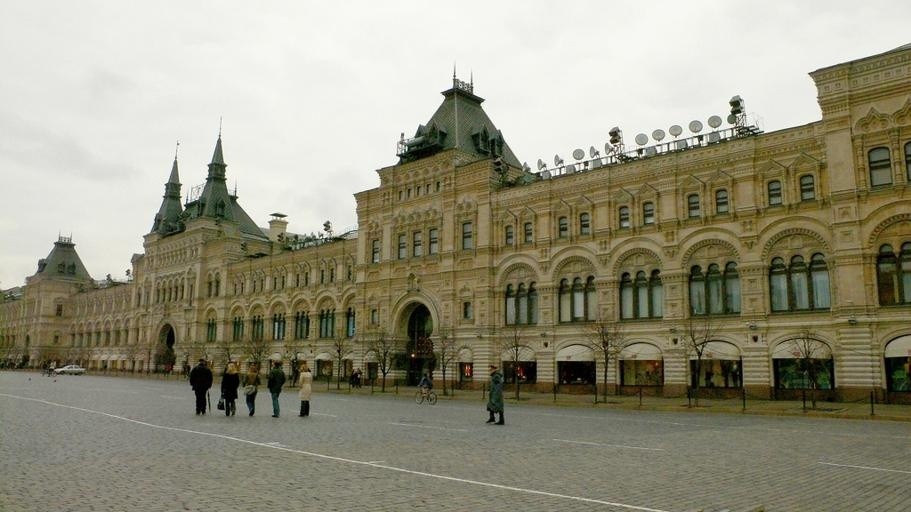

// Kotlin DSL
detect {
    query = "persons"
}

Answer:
[221,362,240,416]
[486,364,505,425]
[354,368,363,389]
[418,373,432,401]
[268,361,286,418]
[297,363,314,417]
[242,365,263,416]
[349,370,356,388]
[190,358,213,416]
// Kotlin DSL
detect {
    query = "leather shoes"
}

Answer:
[486,418,504,425]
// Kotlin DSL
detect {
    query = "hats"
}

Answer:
[487,365,497,370]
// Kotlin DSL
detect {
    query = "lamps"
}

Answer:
[240,241,247,255]
[275,232,283,250]
[728,95,746,137]
[609,126,622,163]
[323,220,332,242]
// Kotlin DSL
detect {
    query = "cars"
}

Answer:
[54,364,86,376]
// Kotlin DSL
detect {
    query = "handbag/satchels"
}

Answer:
[243,385,255,394]
[218,399,224,411]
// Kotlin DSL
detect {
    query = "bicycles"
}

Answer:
[42,367,57,377]
[413,384,438,405]
